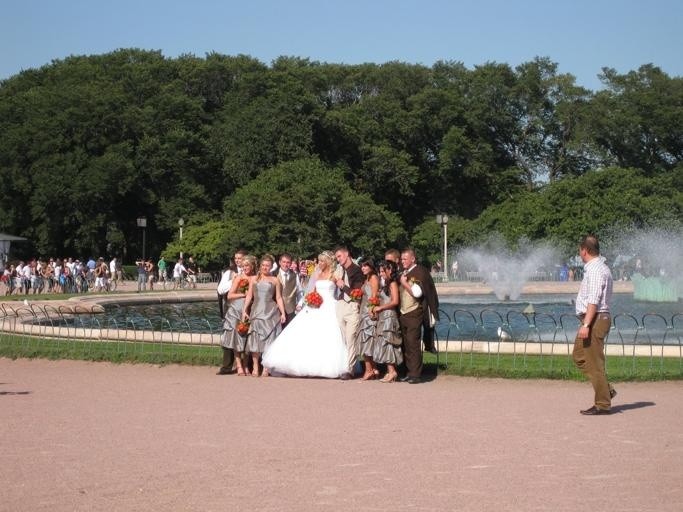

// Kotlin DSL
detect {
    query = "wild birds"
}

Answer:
[497,327,511,343]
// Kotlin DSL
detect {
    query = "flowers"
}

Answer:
[239,279,249,294]
[367,298,380,308]
[350,288,362,301]
[305,291,322,308]
[237,319,251,336]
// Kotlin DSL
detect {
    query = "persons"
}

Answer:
[492,264,498,282]
[572,237,616,415]
[134,256,201,292]
[215,246,458,385]
[618,257,643,281]
[0,256,126,296]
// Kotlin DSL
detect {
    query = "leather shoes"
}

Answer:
[580,390,616,415]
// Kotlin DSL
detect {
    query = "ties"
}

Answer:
[344,270,352,302]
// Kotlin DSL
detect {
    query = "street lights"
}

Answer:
[178,218,184,264]
[435,214,450,283]
[136,215,147,260]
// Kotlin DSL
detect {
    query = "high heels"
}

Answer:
[364,370,419,383]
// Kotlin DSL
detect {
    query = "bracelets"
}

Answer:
[581,323,589,328]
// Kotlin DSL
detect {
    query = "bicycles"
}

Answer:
[163,273,194,291]
